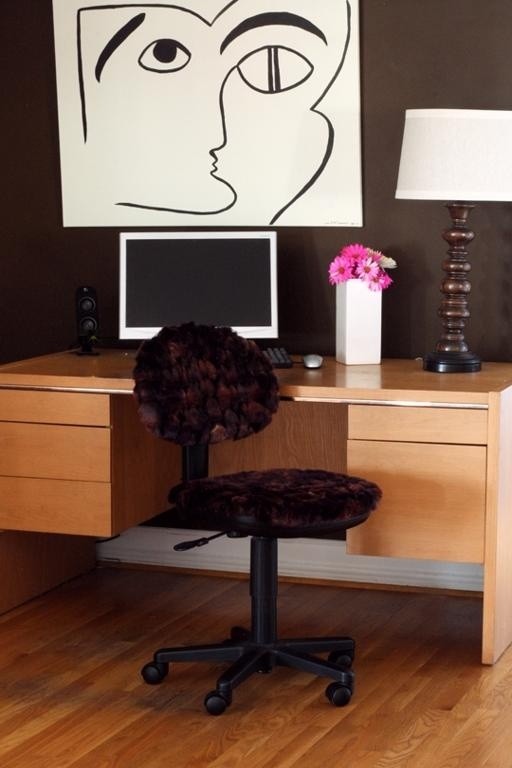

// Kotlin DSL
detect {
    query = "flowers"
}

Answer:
[329,243,396,292]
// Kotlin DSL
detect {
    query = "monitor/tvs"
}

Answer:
[118,232,278,341]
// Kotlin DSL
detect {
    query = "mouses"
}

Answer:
[303,354,323,368]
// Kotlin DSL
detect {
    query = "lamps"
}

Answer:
[394,109,512,374]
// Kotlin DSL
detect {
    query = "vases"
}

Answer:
[335,276,382,365]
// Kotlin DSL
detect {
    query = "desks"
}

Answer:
[0,351,512,666]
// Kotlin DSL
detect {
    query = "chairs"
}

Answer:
[130,322,383,717]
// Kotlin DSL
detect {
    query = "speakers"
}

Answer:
[73,286,99,356]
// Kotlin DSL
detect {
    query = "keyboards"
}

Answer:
[256,345,294,369]
